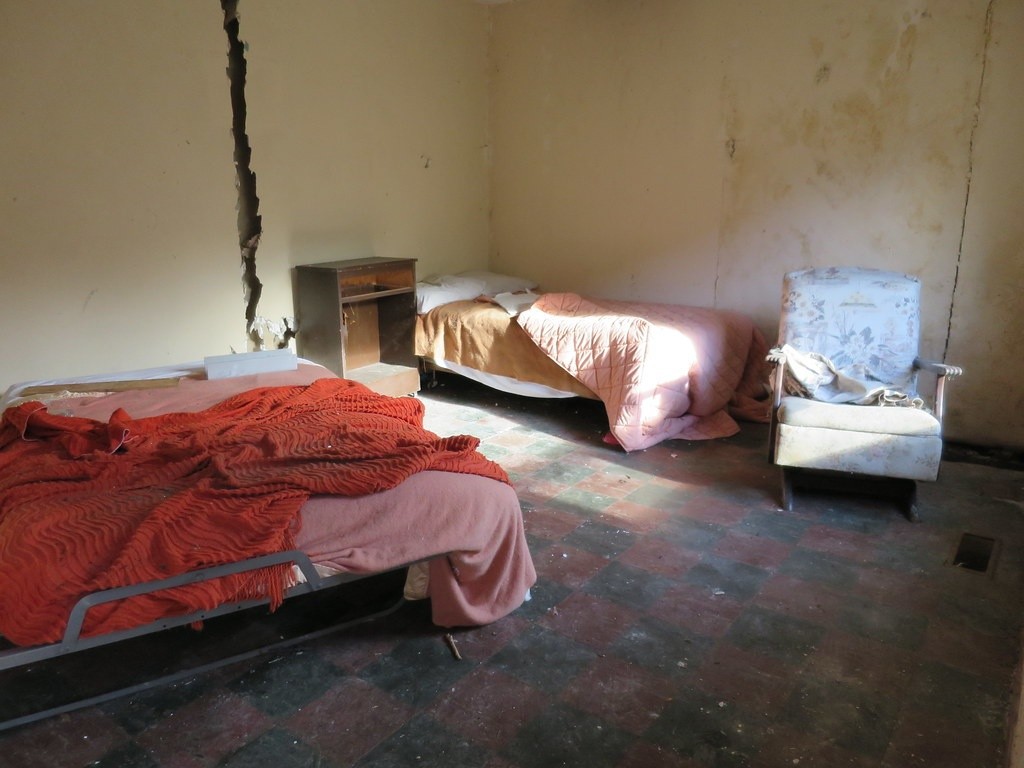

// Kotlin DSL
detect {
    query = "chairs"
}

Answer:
[766,268,963,519]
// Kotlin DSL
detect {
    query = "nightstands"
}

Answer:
[296,255,423,402]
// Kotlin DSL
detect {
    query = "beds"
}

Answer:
[414,291,774,451]
[1,358,537,732]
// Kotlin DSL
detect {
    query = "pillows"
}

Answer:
[417,275,486,316]
[456,270,537,296]
